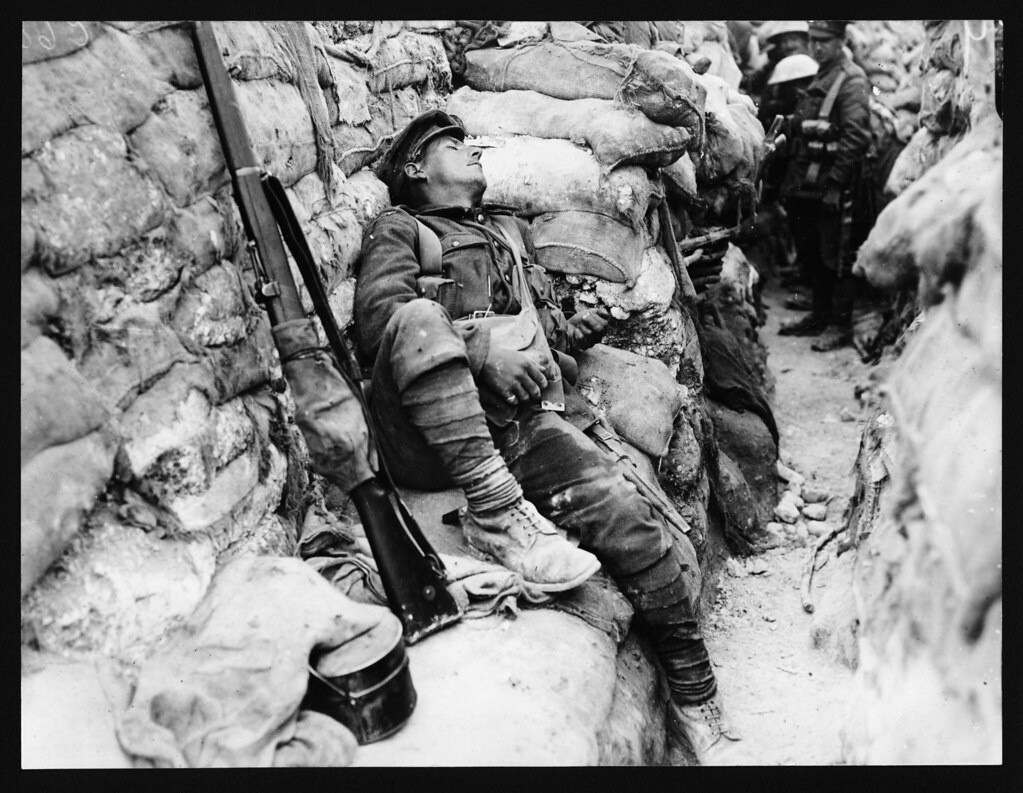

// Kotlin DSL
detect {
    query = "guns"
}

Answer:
[185,19,461,648]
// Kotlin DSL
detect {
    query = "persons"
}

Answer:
[746,19,873,354]
[352,110,753,765]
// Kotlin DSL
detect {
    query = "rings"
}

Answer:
[506,394,516,404]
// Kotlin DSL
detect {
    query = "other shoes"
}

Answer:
[778,309,829,337]
[786,289,815,311]
[810,326,845,351]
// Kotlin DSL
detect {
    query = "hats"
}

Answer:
[375,109,465,189]
[806,18,855,39]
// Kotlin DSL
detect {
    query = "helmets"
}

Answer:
[757,20,809,44]
[768,53,820,85]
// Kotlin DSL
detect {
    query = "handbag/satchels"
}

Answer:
[452,307,558,382]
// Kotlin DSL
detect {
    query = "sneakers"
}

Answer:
[461,497,600,592]
[666,687,751,765]
